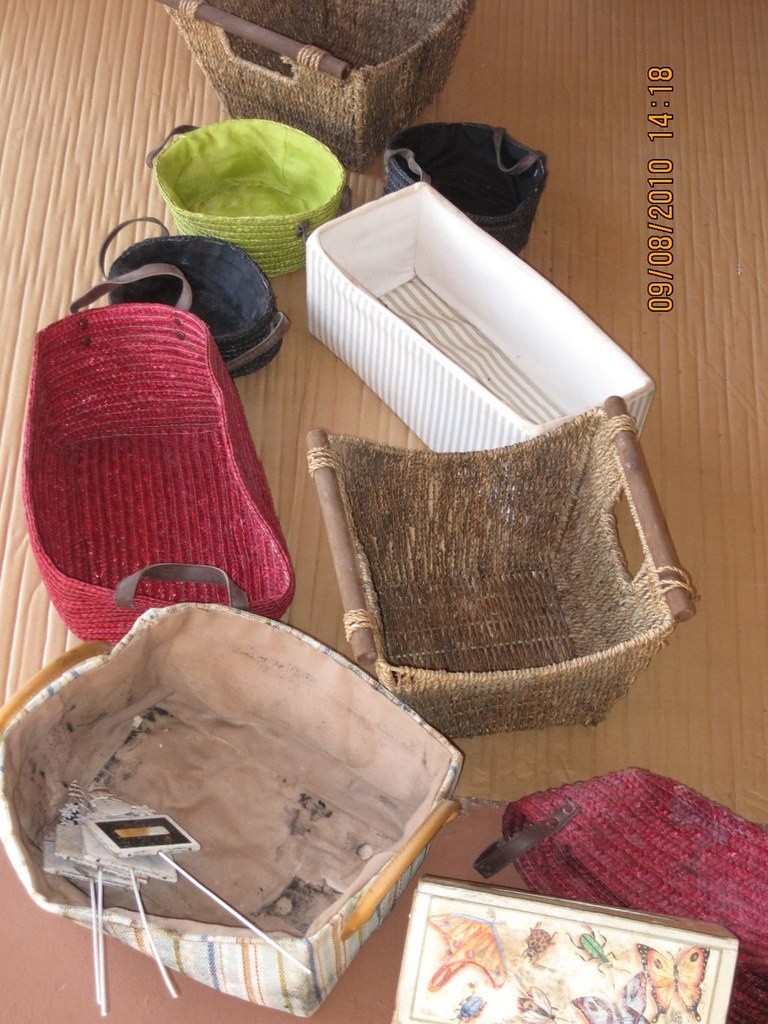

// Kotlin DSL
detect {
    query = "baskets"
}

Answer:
[159,1,473,173]
[22,263,295,643]
[473,762,767,1024]
[0,604,464,1019]
[148,118,353,277]
[99,217,291,378]
[305,396,696,740]
[382,122,548,255]
